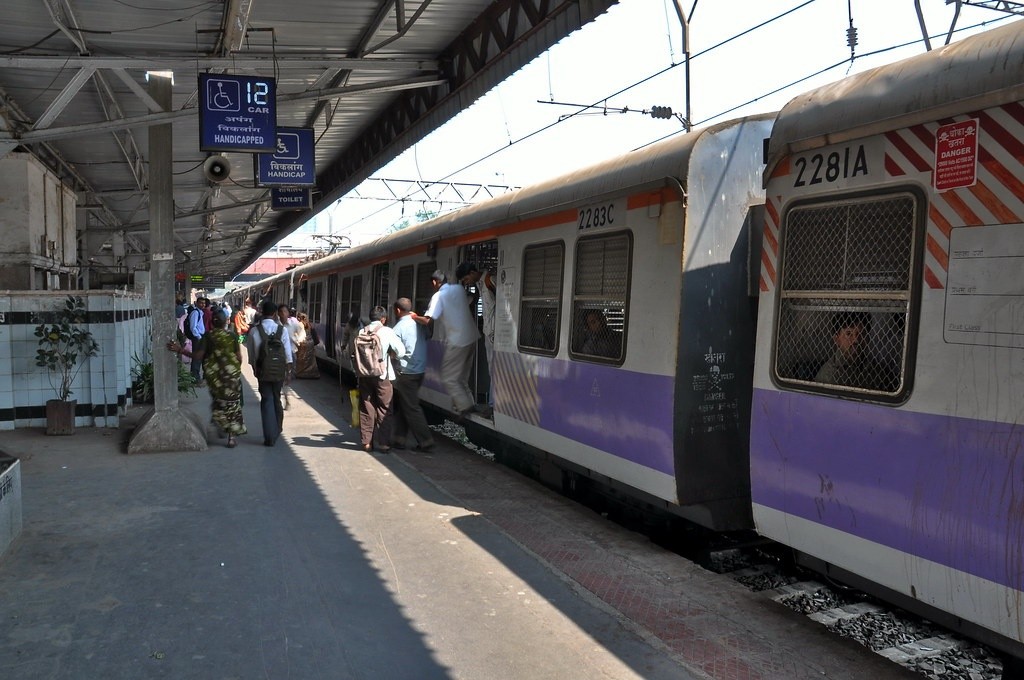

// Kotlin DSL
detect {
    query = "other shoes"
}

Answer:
[379,443,390,454]
[410,443,435,453]
[265,441,275,447]
[457,406,475,414]
[364,441,373,451]
[390,438,406,450]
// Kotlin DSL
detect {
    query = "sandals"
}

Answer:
[229,439,237,446]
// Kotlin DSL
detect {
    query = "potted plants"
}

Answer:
[33,294,101,436]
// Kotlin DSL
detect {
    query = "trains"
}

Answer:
[207,19,1024,663]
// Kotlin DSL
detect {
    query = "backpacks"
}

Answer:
[185,309,201,340]
[354,324,389,377]
[256,324,286,382]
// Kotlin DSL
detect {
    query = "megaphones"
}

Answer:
[204,155,230,183]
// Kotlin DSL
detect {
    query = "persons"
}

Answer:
[166,310,248,448]
[811,310,892,402]
[279,303,306,411]
[578,309,621,359]
[248,303,293,447]
[340,308,366,381]
[176,295,257,389]
[409,269,482,423]
[356,306,406,453]
[454,261,496,417]
[390,298,437,451]
[297,313,321,380]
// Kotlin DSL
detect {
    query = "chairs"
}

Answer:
[778,303,903,377]
